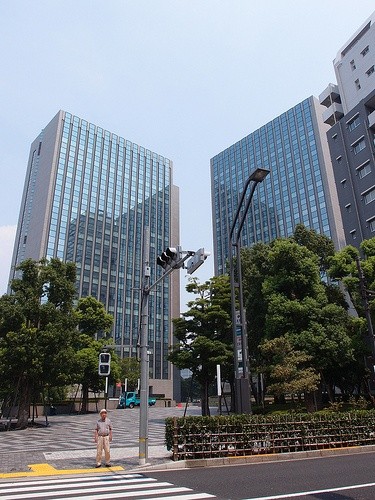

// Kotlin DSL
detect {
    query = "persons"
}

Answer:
[94,409,114,468]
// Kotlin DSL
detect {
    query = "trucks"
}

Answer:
[120,391,156,409]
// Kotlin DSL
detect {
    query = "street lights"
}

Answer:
[229,169,270,414]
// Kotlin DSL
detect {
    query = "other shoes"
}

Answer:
[96,464,101,468]
[105,464,111,467]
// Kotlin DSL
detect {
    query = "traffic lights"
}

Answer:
[157,247,176,269]
[99,352,110,376]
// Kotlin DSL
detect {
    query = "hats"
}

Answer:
[99,408,107,413]
